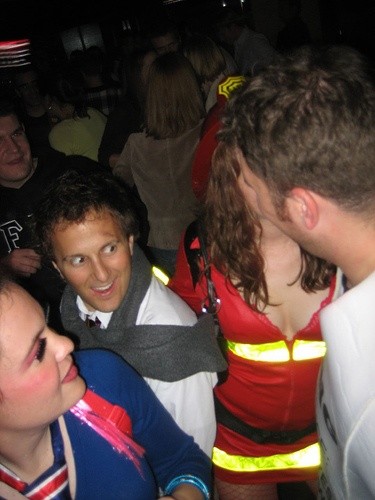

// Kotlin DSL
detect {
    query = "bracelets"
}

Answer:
[165,474,210,500]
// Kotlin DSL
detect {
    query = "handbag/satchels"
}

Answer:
[193,218,228,386]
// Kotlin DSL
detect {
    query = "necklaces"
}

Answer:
[342,273,350,293]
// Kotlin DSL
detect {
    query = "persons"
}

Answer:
[107,9,283,174]
[34,172,228,461]
[166,76,352,500]
[0,274,214,500]
[1,106,67,335]
[222,57,375,500]
[10,60,124,179]
[113,51,206,277]
